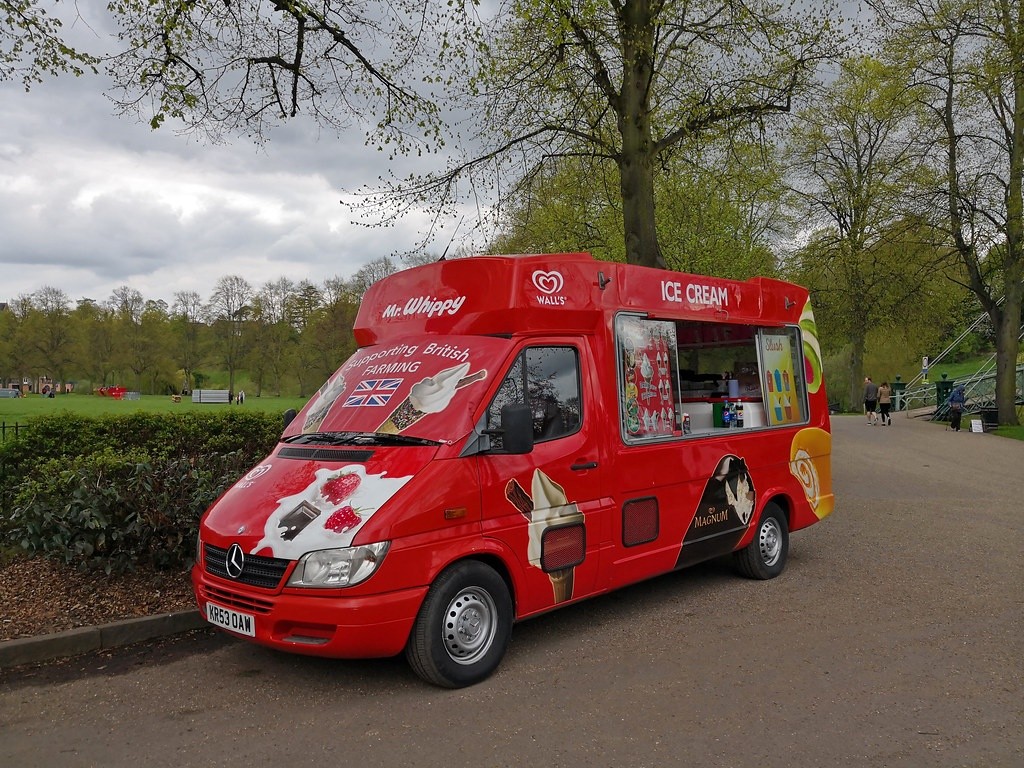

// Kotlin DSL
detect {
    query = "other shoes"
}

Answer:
[950,426,954,431]
[881,423,885,426]
[874,418,878,424]
[867,423,871,426]
[956,429,962,432]
[887,418,891,426]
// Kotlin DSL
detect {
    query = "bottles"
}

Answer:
[736,399,743,428]
[766,369,791,392]
[720,400,732,428]
[675,410,691,435]
[730,402,738,430]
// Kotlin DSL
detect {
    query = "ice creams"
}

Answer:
[640,352,674,431]
[374,361,488,435]
[505,468,585,605]
[302,373,346,433]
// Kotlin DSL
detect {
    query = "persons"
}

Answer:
[15,389,19,398]
[229,390,245,405]
[862,376,878,426]
[948,385,965,432]
[876,380,891,426]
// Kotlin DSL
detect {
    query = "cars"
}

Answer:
[0,388,24,398]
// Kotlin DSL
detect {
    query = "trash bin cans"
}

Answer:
[981,407,998,430]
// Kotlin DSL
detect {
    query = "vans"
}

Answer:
[190,251,837,691]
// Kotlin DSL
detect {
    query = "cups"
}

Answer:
[729,380,738,398]
[784,405,793,420]
[775,407,783,421]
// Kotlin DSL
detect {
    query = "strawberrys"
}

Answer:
[324,501,374,533]
[321,470,361,505]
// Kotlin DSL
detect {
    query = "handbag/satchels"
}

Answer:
[863,403,867,416]
[950,403,961,411]
